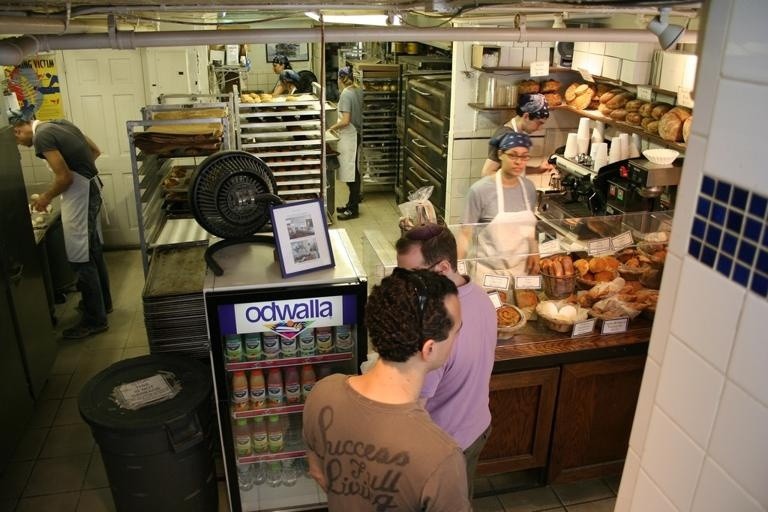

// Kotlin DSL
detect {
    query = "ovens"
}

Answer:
[399,70,452,225]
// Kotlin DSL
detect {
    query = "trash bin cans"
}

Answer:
[78,352,219,512]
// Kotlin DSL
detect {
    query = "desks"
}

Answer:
[25,185,61,325]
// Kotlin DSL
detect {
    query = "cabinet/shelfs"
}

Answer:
[232,81,341,225]
[469,66,694,151]
[363,211,668,500]
[347,63,403,201]
[126,92,236,281]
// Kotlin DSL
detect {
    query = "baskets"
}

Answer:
[496,265,659,340]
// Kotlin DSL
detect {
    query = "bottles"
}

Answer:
[225,327,352,492]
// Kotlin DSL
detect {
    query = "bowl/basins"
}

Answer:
[643,148,679,165]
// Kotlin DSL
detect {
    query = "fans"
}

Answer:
[187,150,286,277]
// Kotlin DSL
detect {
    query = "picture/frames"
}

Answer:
[265,42,309,63]
[269,197,336,279]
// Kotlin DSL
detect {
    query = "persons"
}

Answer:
[305,270,480,512]
[9,107,114,342]
[456,135,542,286]
[327,67,367,220]
[482,94,555,182]
[278,67,306,96]
[385,223,492,507]
[270,53,289,95]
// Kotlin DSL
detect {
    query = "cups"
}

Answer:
[564,116,641,171]
[484,78,517,108]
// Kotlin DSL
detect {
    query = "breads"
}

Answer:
[518,77,563,110]
[237,91,276,106]
[565,81,693,146]
[285,96,298,102]
[480,239,668,340]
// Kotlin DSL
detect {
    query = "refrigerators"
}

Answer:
[200,227,367,511]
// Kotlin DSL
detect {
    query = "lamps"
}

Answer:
[647,7,685,51]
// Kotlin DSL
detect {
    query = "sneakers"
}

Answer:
[62,324,109,339]
[78,299,114,314]
[336,206,346,211]
[337,210,358,220]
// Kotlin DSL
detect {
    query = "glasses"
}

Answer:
[395,268,427,351]
[504,153,531,161]
